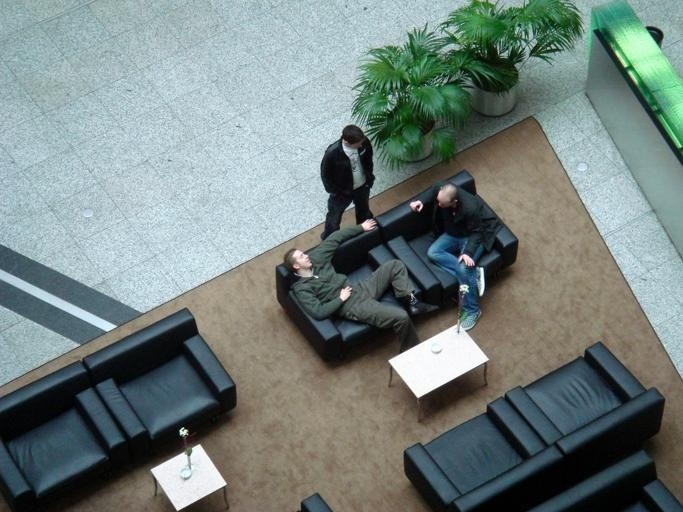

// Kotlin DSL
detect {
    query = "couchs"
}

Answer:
[81,307,237,470]
[0,359,139,509]
[275,223,421,366]
[505,342,664,480]
[531,450,683,511]
[376,170,521,311]
[401,398,564,512]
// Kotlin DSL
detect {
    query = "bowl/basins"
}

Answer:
[431,344,443,354]
[180,468,192,480]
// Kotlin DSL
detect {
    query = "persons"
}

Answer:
[283,219,440,352]
[409,182,502,330]
[320,124,375,242]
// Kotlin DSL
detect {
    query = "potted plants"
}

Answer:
[350,26,467,173]
[442,0,588,119]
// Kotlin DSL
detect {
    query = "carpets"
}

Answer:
[1,115,681,510]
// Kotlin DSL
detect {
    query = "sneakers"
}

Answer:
[410,302,439,316]
[321,232,328,239]
[400,350,403,354]
[458,310,482,330]
[476,267,486,297]
